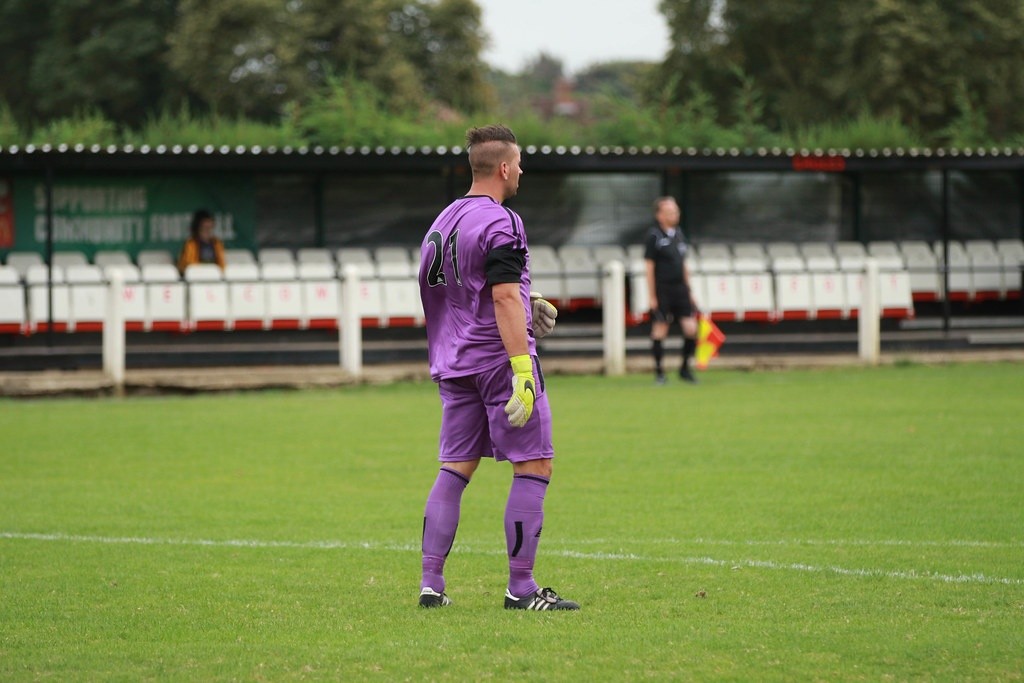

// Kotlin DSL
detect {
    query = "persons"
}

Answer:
[643,193,700,381]
[419,123,583,610]
[182,210,224,279]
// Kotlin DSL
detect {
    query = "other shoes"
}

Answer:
[658,373,667,385]
[680,370,696,386]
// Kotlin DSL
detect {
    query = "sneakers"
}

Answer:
[419,587,452,608]
[503,586,580,611]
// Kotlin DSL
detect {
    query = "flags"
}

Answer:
[695,307,724,373]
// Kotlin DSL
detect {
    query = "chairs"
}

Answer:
[0,238,1023,332]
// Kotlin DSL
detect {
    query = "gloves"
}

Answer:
[504,358,536,428]
[530,292,558,339]
